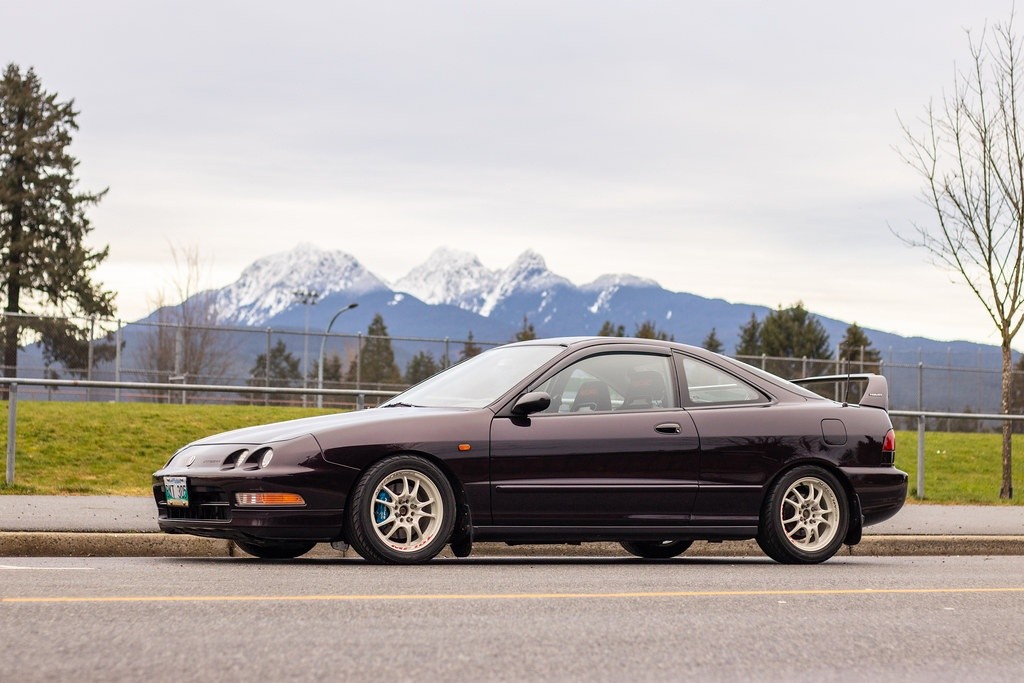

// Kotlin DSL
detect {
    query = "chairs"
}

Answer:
[568,379,612,412]
[616,371,668,411]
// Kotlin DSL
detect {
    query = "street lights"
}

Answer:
[322,303,359,406]
[293,290,320,405]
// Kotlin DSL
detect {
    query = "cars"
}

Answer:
[152,335,909,563]
